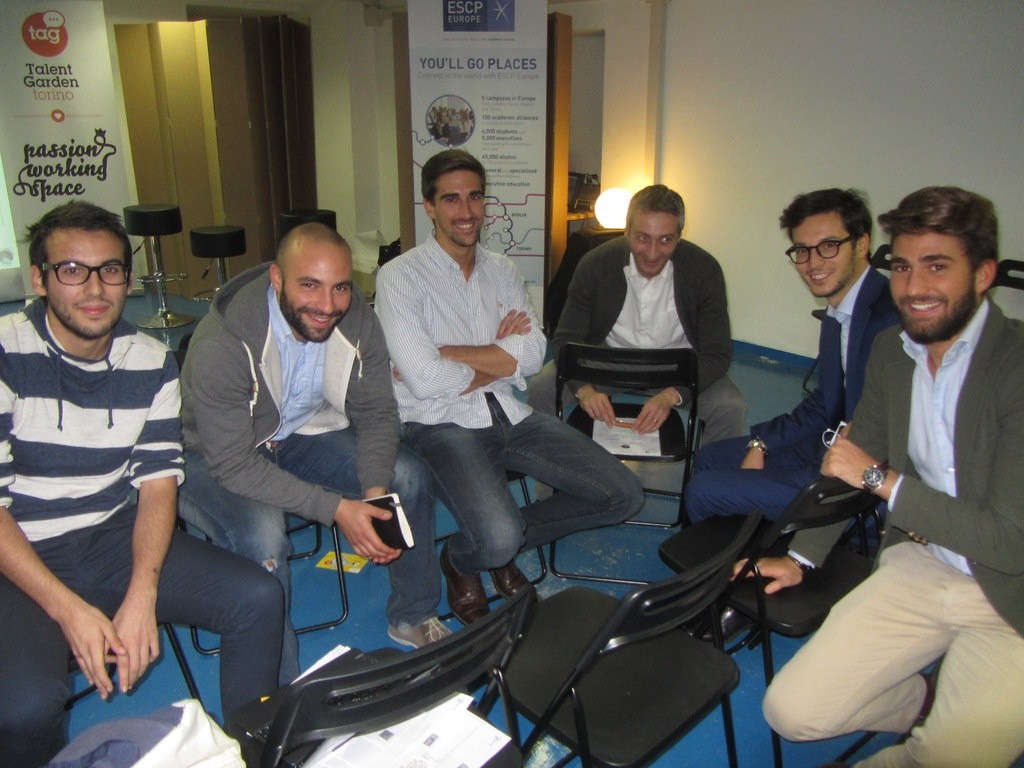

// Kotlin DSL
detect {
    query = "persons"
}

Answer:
[426,106,475,142]
[178,223,453,688]
[526,183,752,498]
[685,188,901,645]
[374,149,644,627]
[761,186,1024,768]
[0,202,285,768]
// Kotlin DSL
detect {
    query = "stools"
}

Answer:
[280,209,338,238]
[68,618,204,710]
[124,205,195,328]
[190,225,246,287]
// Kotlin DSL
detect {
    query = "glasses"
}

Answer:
[785,232,860,264]
[41,261,129,286]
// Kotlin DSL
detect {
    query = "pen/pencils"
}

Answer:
[613,421,633,427]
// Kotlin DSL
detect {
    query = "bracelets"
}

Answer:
[788,555,808,573]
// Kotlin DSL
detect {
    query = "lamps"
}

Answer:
[594,187,639,230]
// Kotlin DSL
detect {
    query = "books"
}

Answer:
[363,493,414,549]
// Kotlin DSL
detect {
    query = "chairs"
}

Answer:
[178,339,886,768]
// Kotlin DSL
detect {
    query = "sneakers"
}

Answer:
[388,616,452,650]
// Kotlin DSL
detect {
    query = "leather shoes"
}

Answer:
[439,537,490,625]
[488,560,530,599]
[688,607,758,644]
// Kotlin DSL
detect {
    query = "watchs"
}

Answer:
[746,436,768,454]
[862,463,890,493]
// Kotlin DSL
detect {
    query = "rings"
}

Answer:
[653,419,657,422]
